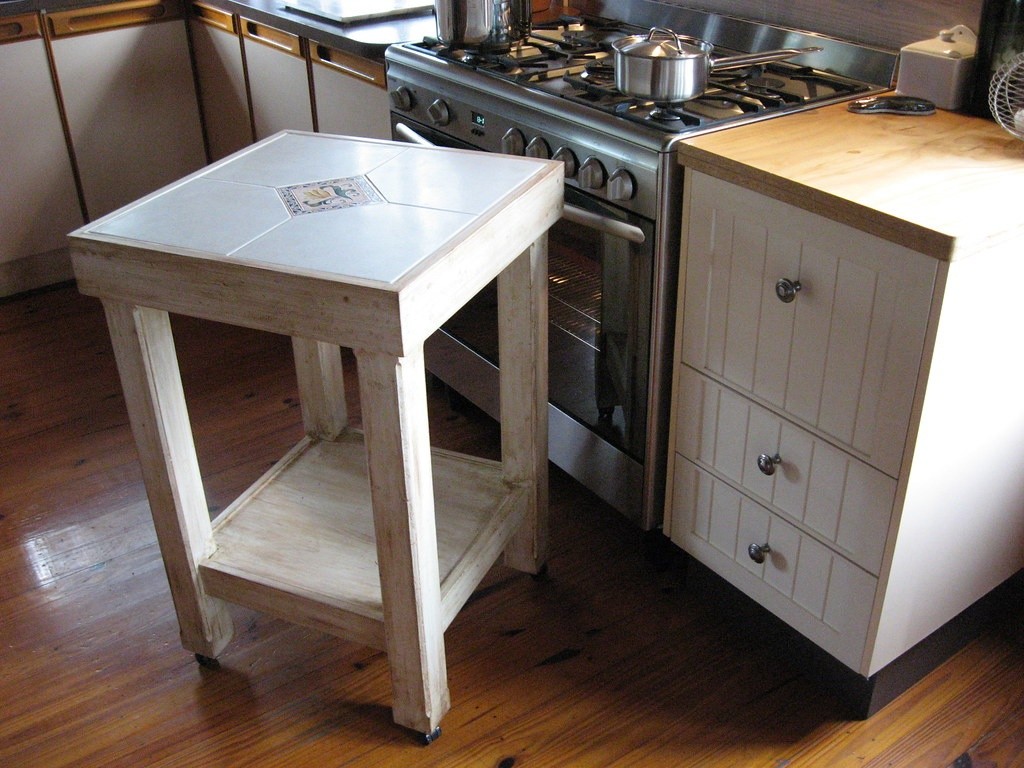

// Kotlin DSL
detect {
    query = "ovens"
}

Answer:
[385,109,657,527]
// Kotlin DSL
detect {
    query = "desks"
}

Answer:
[63,129,564,746]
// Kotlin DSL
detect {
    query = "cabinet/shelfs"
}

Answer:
[661,91,1024,723]
[0,0,209,299]
[189,0,394,164]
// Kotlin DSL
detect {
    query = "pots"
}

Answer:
[433,0,532,45]
[609,25,824,101]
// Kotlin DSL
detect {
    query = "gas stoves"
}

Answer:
[386,0,900,220]
